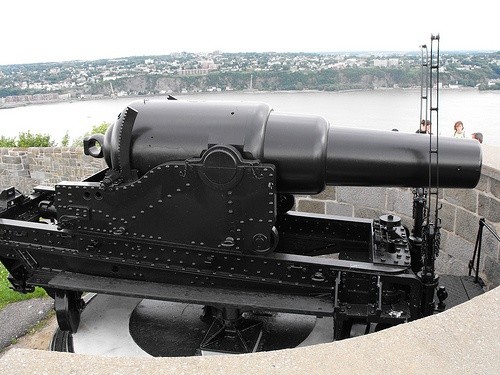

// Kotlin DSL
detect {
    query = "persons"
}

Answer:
[450,120,467,139]
[472,132,484,144]
[417,119,435,135]
